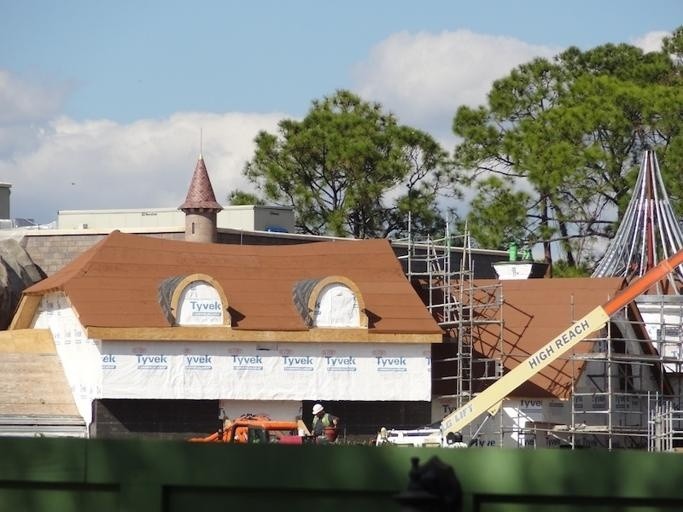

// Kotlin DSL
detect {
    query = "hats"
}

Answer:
[312,404,324,414]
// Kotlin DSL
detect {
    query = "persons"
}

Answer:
[311,404,340,437]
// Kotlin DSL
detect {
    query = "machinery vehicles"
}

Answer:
[186,248,683,445]
